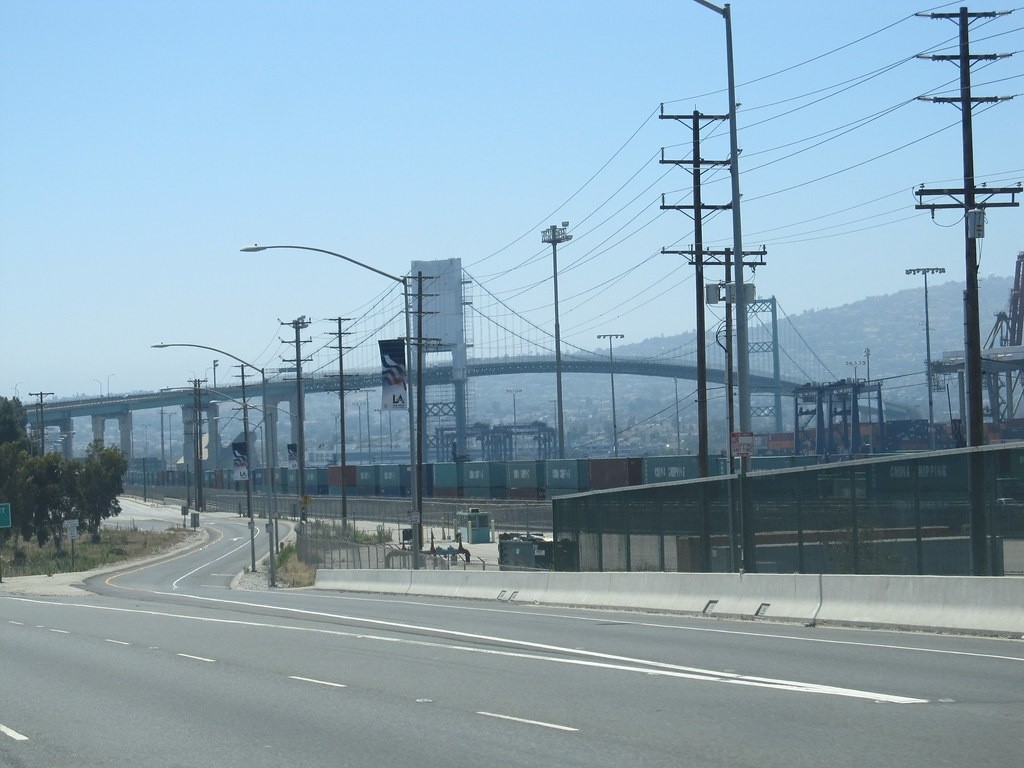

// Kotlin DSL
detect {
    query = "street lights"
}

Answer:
[8,333,886,552]
[240,243,422,573]
[905,267,946,455]
[541,219,573,460]
